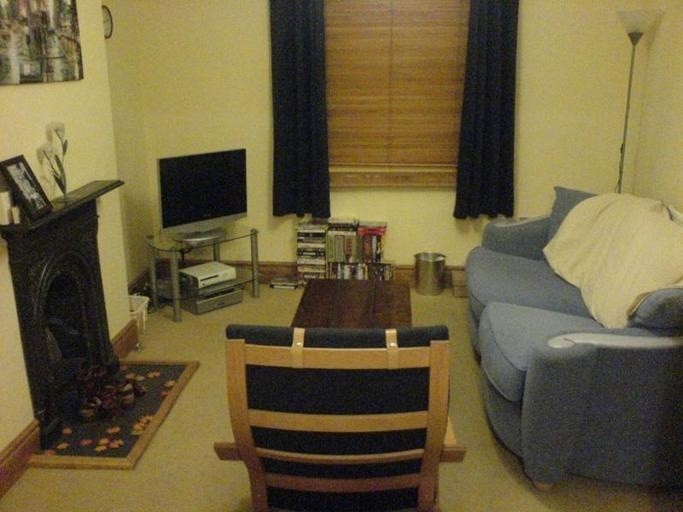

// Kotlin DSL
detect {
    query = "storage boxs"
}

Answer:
[127,295,150,329]
[451,270,468,298]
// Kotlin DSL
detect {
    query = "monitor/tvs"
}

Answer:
[156,147,247,242]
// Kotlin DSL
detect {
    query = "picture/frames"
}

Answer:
[0,154,53,220]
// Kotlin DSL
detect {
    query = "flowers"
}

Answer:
[37,122,70,198]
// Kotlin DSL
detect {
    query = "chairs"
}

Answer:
[213,325,463,512]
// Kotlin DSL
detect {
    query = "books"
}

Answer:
[268,217,395,290]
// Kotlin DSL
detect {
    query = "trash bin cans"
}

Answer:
[416,254,444,296]
[130,295,148,334]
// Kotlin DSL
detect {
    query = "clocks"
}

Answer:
[102,4,113,38]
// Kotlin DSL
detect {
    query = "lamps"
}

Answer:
[615,6,659,191]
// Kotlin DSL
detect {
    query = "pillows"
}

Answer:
[542,186,595,259]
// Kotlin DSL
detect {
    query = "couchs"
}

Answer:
[465,193,683,491]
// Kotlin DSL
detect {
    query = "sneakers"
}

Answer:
[78,374,146,423]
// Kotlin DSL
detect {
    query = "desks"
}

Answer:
[144,223,261,321]
